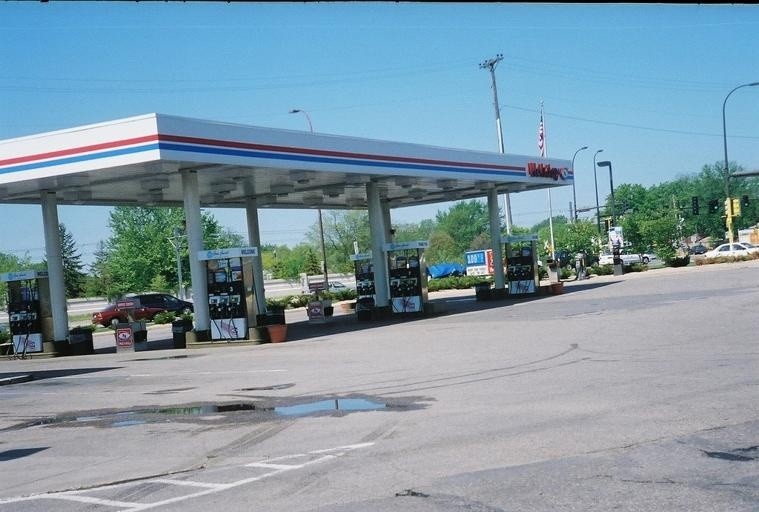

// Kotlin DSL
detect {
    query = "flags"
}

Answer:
[536,105,545,156]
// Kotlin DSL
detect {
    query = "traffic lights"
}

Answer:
[734,200,741,217]
[692,196,699,216]
[742,196,750,208]
[724,198,731,219]
[710,200,719,214]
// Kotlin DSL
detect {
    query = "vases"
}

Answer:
[267,324,288,343]
[551,282,563,294]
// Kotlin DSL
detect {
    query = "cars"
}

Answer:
[303,280,352,295]
[90,291,193,326]
[547,243,759,272]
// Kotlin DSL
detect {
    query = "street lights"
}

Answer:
[570,144,605,247]
[290,107,331,302]
[597,160,616,226]
